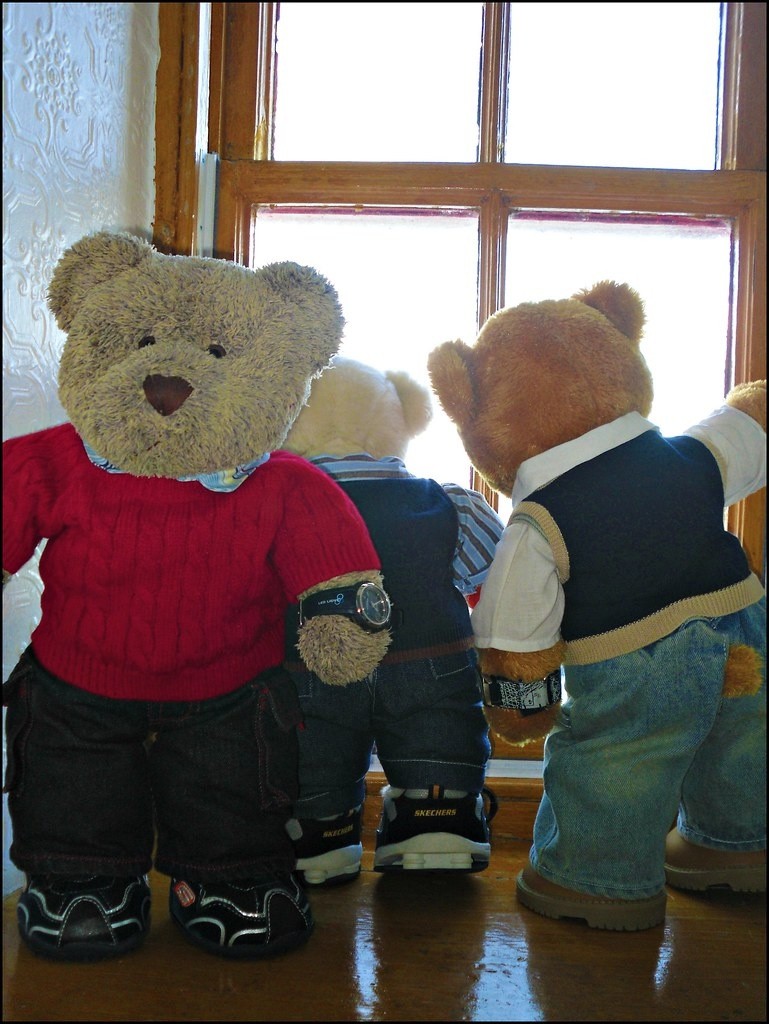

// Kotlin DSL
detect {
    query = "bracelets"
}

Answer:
[482,667,562,717]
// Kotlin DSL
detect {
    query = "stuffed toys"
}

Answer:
[1,230,391,960]
[277,359,506,888]
[426,278,767,930]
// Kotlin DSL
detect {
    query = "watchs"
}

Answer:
[297,581,393,635]
